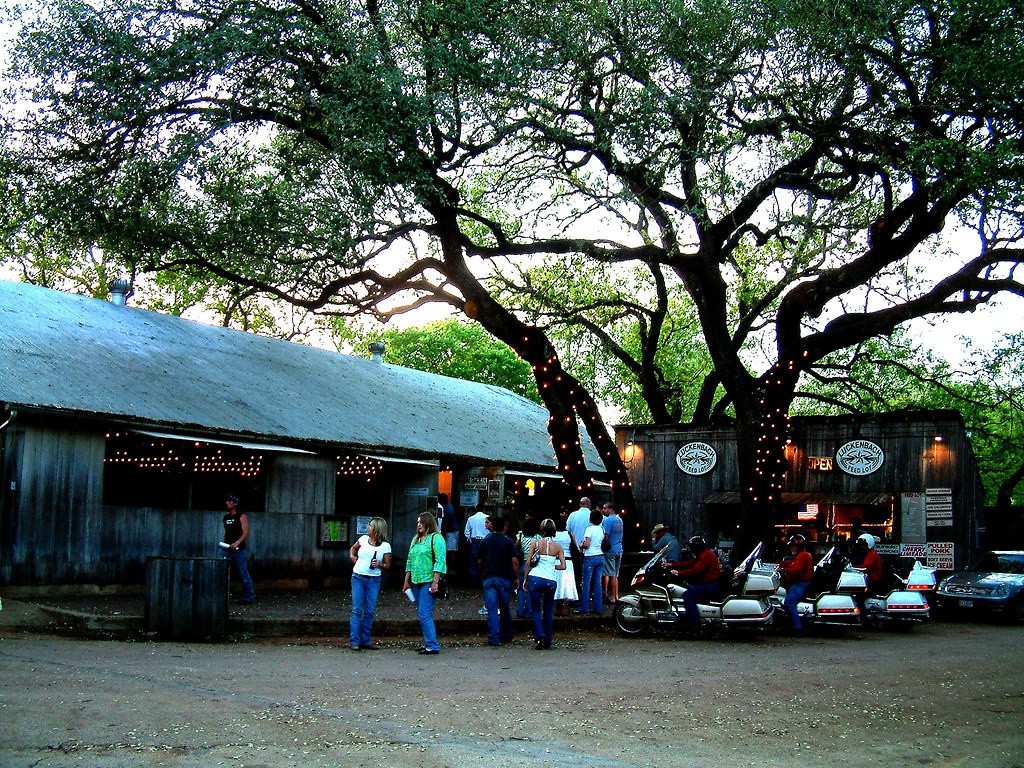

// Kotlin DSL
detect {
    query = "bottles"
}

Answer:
[369,551,377,570]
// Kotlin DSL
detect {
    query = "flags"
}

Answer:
[733,542,765,578]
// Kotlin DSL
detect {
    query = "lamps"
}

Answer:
[628,437,634,446]
[934,434,942,440]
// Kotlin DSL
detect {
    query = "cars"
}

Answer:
[936,551,1024,626]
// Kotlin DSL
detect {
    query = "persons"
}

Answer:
[662,536,722,624]
[523,519,566,650]
[402,512,447,654]
[853,533,882,616]
[651,524,681,562]
[438,493,623,617]
[478,516,520,646]
[215,492,255,603]
[350,517,391,649]
[776,534,813,636]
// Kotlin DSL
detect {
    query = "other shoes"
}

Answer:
[414,645,425,651]
[602,597,619,604]
[591,610,603,616]
[352,645,359,650]
[535,637,550,650]
[360,644,380,650]
[576,610,590,616]
[419,647,438,654]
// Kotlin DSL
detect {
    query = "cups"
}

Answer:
[405,588,416,603]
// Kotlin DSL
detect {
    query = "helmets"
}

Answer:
[856,533,876,550]
[685,536,706,556]
[787,535,806,551]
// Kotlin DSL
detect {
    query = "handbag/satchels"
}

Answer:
[530,542,540,568]
[435,577,449,600]
[601,525,611,552]
[516,533,523,567]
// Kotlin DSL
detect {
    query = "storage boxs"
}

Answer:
[721,568,780,624]
[817,572,866,617]
[886,568,936,620]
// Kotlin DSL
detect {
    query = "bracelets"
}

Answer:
[236,541,240,544]
[384,564,385,567]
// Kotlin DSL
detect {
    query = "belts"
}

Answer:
[555,557,573,560]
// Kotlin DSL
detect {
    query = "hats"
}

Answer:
[226,494,240,505]
[651,524,669,536]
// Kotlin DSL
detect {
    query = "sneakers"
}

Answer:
[497,608,501,615]
[478,605,488,615]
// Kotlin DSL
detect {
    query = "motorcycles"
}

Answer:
[813,546,937,633]
[612,542,782,642]
[722,542,868,637]
